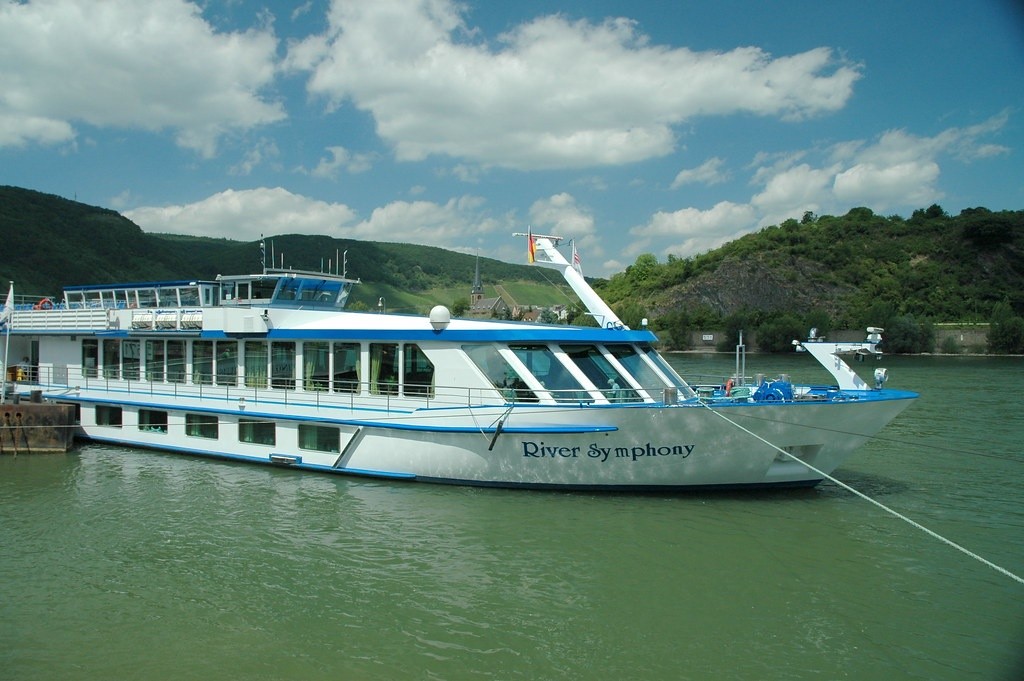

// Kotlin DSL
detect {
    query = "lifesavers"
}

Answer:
[724,378,735,396]
[40,299,53,309]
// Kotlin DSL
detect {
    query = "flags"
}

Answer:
[0,284,15,324]
[572,242,584,280]
[527,227,536,264]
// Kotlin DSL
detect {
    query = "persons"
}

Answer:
[18,356,32,381]
[607,379,620,399]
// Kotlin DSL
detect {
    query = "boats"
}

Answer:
[0,233,920,486]
